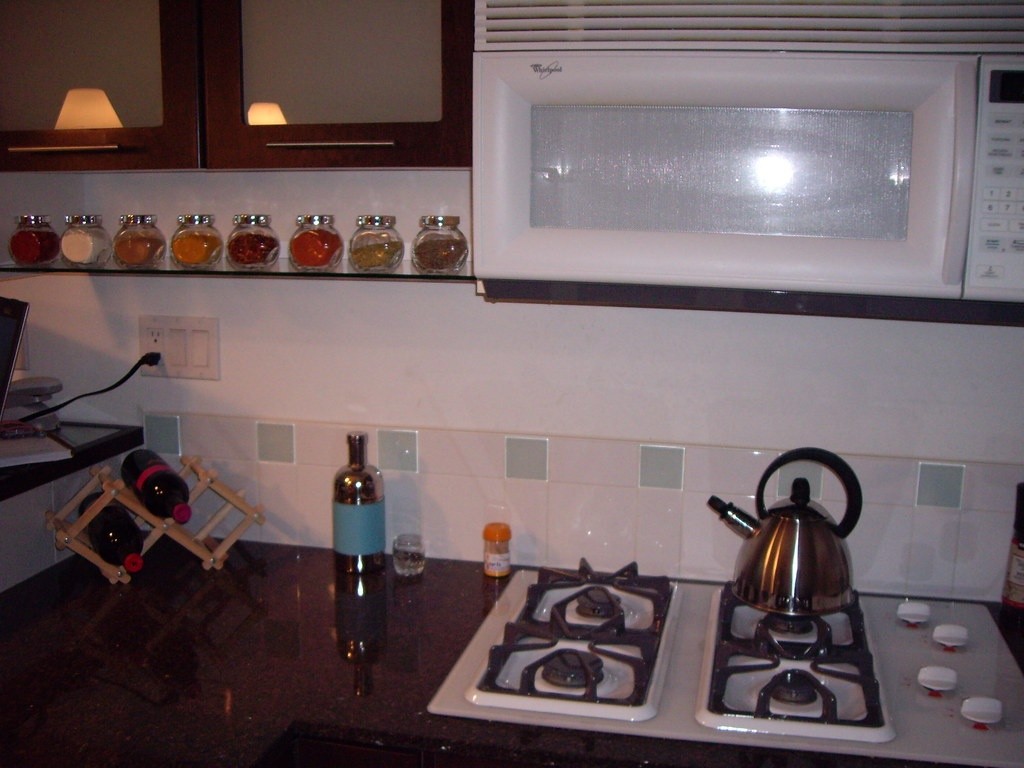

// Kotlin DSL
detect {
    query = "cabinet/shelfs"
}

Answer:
[0,0,472,173]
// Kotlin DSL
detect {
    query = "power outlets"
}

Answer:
[139,315,220,381]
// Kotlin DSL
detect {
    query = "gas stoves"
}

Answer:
[426,557,1024,765]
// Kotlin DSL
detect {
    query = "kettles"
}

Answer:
[709,441,862,615]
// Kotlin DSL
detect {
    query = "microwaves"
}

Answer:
[472,1,1024,303]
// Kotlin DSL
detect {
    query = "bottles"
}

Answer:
[411,215,468,274]
[484,522,512,576]
[332,432,384,573]
[121,449,191,523]
[112,215,165,269]
[79,493,143,572]
[349,215,404,272]
[61,214,112,268]
[170,215,222,270]
[226,215,280,270]
[289,214,345,271]
[9,215,61,266]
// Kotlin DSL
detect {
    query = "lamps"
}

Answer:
[248,102,287,125]
[54,89,123,128]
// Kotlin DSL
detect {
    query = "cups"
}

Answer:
[393,534,424,574]
